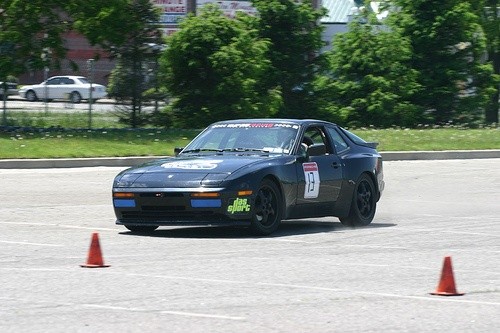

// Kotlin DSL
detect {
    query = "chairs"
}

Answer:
[292,136,314,151]
[235,136,264,148]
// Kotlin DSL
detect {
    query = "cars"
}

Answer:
[112,119,385,238]
[0,79,19,99]
[18,76,107,103]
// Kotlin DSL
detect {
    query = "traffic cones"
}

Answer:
[78,232,112,268]
[429,255,465,296]
